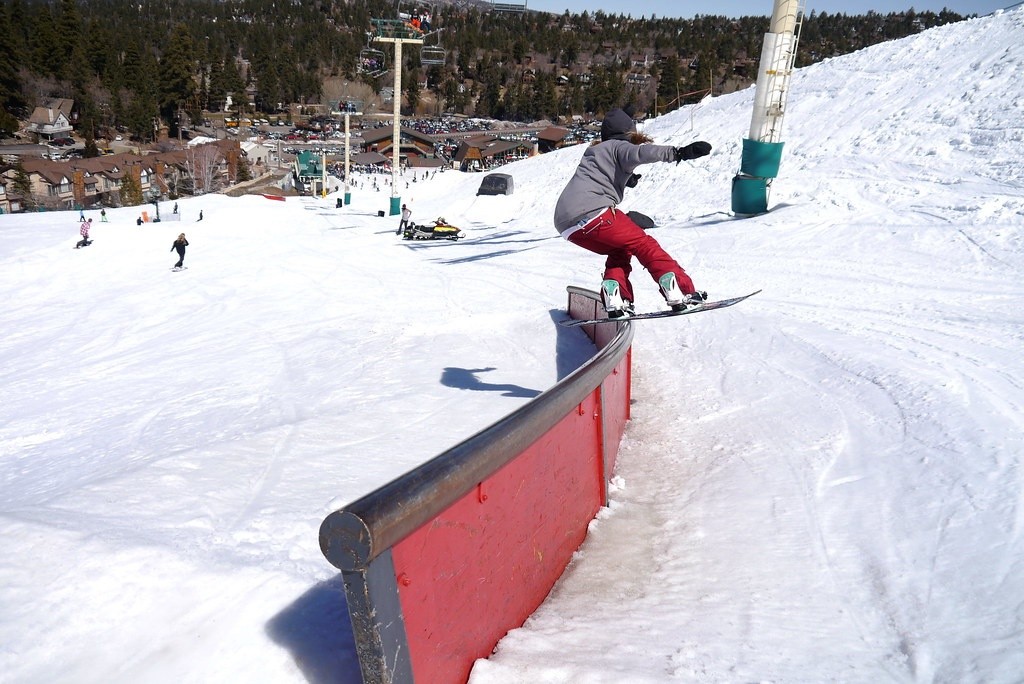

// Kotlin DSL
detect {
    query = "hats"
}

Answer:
[601,109,637,143]
[403,204,406,207]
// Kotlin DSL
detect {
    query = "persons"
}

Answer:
[199,210,203,221]
[173,202,178,214]
[76,218,92,248]
[362,56,382,72]
[296,150,458,194]
[101,208,108,222]
[171,233,189,269]
[420,9,433,33]
[137,217,144,225]
[411,8,420,31]
[79,209,86,222]
[397,204,412,235]
[554,106,712,321]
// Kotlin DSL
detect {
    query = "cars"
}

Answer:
[177,113,602,175]
[49,138,76,145]
[42,152,82,161]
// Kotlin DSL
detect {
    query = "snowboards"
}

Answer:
[556,288,763,328]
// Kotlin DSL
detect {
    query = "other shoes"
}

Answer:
[602,280,635,319]
[659,272,708,311]
[396,231,401,235]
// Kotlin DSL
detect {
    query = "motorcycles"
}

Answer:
[405,218,466,241]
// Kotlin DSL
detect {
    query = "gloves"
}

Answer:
[625,174,642,188]
[676,141,712,166]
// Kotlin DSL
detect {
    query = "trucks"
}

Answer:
[116,135,123,141]
[102,148,115,156]
[97,148,107,156]
